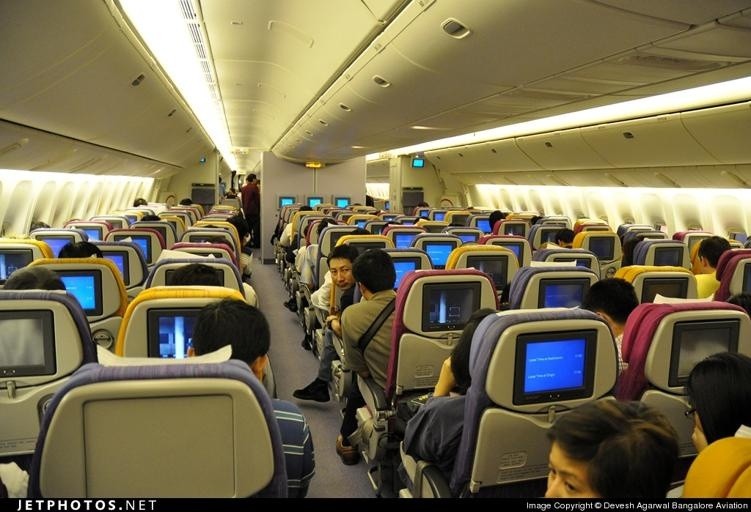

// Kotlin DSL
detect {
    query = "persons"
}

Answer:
[59,241,103,259]
[239,175,243,191]
[582,278,639,377]
[694,236,733,298]
[617,223,627,236]
[499,276,517,311]
[203,236,260,308]
[392,307,503,498]
[366,186,374,207]
[219,177,225,200]
[411,201,428,216]
[477,210,506,245]
[743,234,751,250]
[283,216,315,313]
[242,174,261,248]
[180,198,195,205]
[166,195,177,207]
[554,228,575,249]
[279,205,313,277]
[622,237,641,267]
[141,214,161,221]
[226,215,254,281]
[170,262,220,288]
[298,218,339,314]
[134,197,147,208]
[227,187,237,199]
[528,215,544,229]
[293,244,360,402]
[301,227,371,351]
[188,298,316,496]
[544,399,680,500]
[682,351,751,499]
[1,264,67,500]
[335,247,397,466]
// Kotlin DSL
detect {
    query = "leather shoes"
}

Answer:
[300,334,315,350]
[283,298,299,313]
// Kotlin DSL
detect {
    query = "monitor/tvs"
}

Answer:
[330,232,350,249]
[590,237,613,261]
[689,237,705,253]
[453,214,470,226]
[420,210,431,217]
[549,223,566,227]
[454,233,479,244]
[537,279,592,316]
[421,240,456,268]
[335,198,350,209]
[0,249,33,284]
[513,332,602,401]
[277,195,297,209]
[145,307,207,361]
[639,278,688,306]
[99,250,130,285]
[164,268,223,286]
[189,235,227,242]
[554,257,591,270]
[403,222,413,225]
[385,216,394,221]
[136,225,167,247]
[370,225,384,234]
[1,307,58,376]
[105,220,123,231]
[389,257,421,290]
[47,268,102,318]
[411,157,425,168]
[38,239,70,257]
[126,216,138,223]
[467,256,507,291]
[73,223,105,242]
[114,233,153,265]
[342,215,350,221]
[427,227,445,233]
[505,223,526,238]
[475,218,494,234]
[350,243,385,256]
[434,212,445,220]
[587,228,608,231]
[424,281,483,330]
[385,202,390,209]
[393,232,416,250]
[494,241,523,266]
[305,195,325,210]
[539,229,560,244]
[669,322,739,378]
[64,316,64,317]
[653,246,684,267]
[355,220,368,229]
[200,158,206,163]
[641,235,663,239]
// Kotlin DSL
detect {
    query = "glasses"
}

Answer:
[681,404,700,422]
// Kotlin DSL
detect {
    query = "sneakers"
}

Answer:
[293,381,331,406]
[336,434,363,466]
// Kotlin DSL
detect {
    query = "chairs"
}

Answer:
[128,221,177,249]
[279,210,323,281]
[393,307,620,500]
[378,211,403,222]
[142,257,247,298]
[78,239,149,301]
[507,263,599,313]
[314,203,335,211]
[428,209,448,221]
[441,223,484,245]
[350,267,501,496]
[531,248,603,276]
[469,216,491,232]
[411,206,431,219]
[179,227,241,260]
[126,214,140,224]
[476,233,531,269]
[408,231,463,270]
[346,213,380,226]
[536,218,571,230]
[296,225,371,328]
[383,222,425,250]
[572,216,611,236]
[1,234,54,287]
[444,243,518,298]
[28,225,89,257]
[351,206,378,214]
[89,215,129,232]
[571,229,624,280]
[362,220,395,236]
[109,284,249,371]
[527,224,568,251]
[613,264,698,305]
[191,219,241,256]
[613,299,751,459]
[201,204,237,219]
[321,247,434,422]
[167,242,240,269]
[320,206,340,214]
[21,256,128,363]
[157,209,191,228]
[335,212,353,221]
[328,209,352,217]
[417,221,447,233]
[442,208,472,227]
[288,214,333,302]
[158,215,185,236]
[22,361,290,501]
[307,233,396,361]
[616,222,655,236]
[491,215,530,240]
[0,288,98,468]
[673,230,712,264]
[394,216,420,225]
[632,238,691,269]
[63,219,113,243]
[104,227,164,267]
[289,219,349,300]
[728,237,744,248]
[113,207,155,216]
[707,247,751,306]
